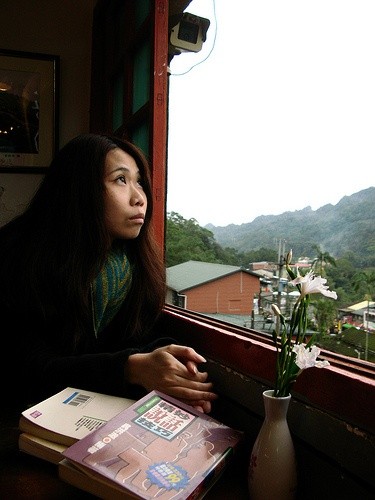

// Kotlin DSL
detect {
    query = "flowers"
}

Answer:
[254,244,338,398]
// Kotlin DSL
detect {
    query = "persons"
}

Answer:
[0,131,218,470]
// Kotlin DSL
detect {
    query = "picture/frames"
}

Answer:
[0,49,61,175]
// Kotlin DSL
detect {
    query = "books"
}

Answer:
[18,386,245,500]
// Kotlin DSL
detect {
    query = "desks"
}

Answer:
[0,416,250,500]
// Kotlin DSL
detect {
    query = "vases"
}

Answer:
[246,390,298,500]
